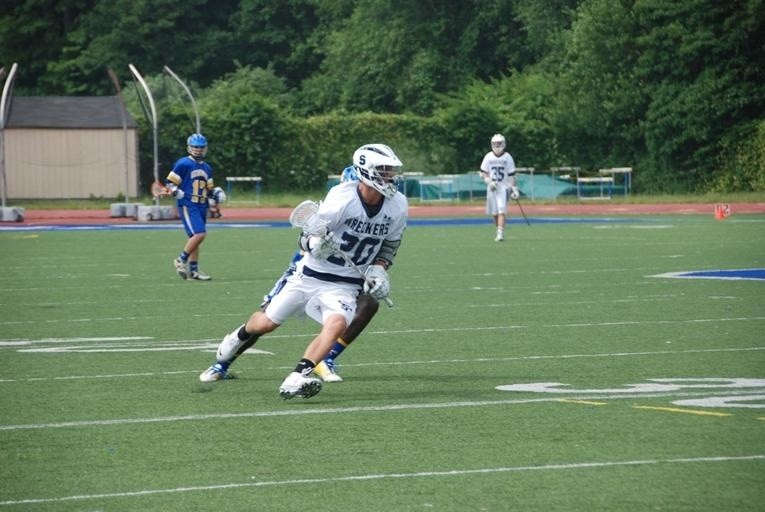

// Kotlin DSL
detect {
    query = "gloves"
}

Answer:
[484,177,498,191]
[213,187,226,203]
[363,264,390,299]
[303,232,341,259]
[510,187,519,200]
[172,189,185,200]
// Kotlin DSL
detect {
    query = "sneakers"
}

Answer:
[189,272,211,280]
[199,323,342,399]
[494,226,505,241]
[174,258,187,280]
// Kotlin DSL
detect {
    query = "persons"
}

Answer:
[215,142,409,399]
[199,165,379,383]
[165,134,226,282]
[479,134,520,242]
[208,187,226,219]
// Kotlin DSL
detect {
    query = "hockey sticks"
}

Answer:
[151,179,219,201]
[290,200,394,308]
[501,176,530,225]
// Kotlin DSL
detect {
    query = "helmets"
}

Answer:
[352,144,403,198]
[342,167,359,183]
[187,133,208,158]
[491,134,506,154]
